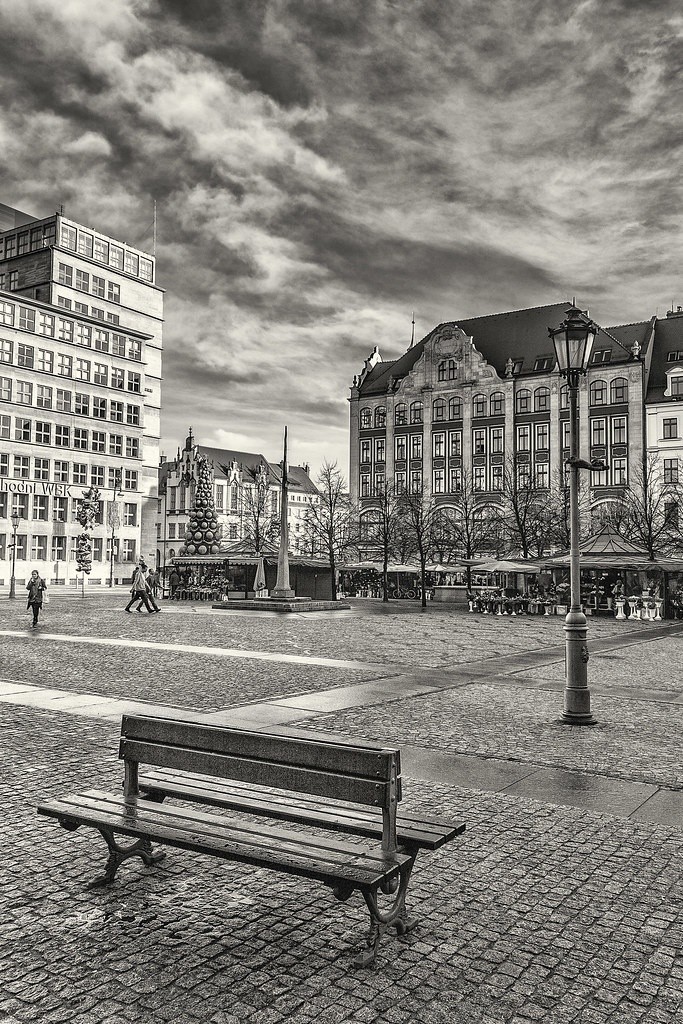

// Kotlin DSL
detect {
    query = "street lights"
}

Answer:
[7,510,24,601]
[546,298,610,727]
[108,485,124,588]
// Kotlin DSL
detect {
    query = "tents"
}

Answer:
[383,557,541,593]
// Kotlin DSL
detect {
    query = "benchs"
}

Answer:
[122,713,466,936]
[37,714,413,970]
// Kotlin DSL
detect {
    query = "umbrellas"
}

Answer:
[253,557,265,593]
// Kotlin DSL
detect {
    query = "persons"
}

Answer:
[125,564,181,614]
[26,570,47,627]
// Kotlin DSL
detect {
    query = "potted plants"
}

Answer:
[642,597,654,619]
[627,598,637,620]
[614,600,625,620]
[614,596,627,619]
[648,603,656,621]
[654,598,664,620]
[555,602,570,616]
[634,603,643,621]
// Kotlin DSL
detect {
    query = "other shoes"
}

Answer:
[125,609,132,613]
[136,607,142,612]
[33,619,38,624]
[149,609,155,613]
[156,609,161,612]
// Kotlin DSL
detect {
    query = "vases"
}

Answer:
[544,606,550,616]
[483,602,489,614]
[673,609,678,620]
[606,597,612,610]
[511,605,516,616]
[497,604,503,616]
[551,605,556,615]
[593,596,602,610]
[469,601,473,612]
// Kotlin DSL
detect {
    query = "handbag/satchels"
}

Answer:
[41,588,49,604]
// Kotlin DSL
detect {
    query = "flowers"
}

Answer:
[466,593,475,603]
[542,602,551,606]
[556,583,570,593]
[669,599,681,611]
[605,591,614,598]
[475,589,542,605]
[591,590,605,598]
[547,598,558,605]
